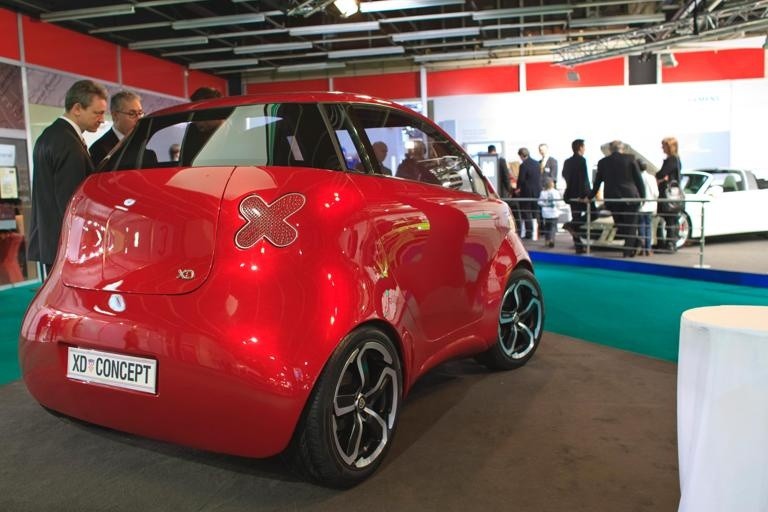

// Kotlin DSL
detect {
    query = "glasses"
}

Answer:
[121,110,144,117]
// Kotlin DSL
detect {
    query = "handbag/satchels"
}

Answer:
[664,180,685,212]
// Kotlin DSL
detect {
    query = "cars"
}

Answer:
[574,169,768,252]
[18,90,544,489]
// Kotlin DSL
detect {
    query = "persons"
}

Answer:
[584,141,646,257]
[168,143,181,162]
[562,140,599,253]
[636,159,659,256]
[516,144,559,247]
[656,140,682,253]
[360,142,392,176]
[88,91,158,166]
[26,80,108,277]
[395,140,445,183]
[488,145,512,198]
[183,88,237,161]
